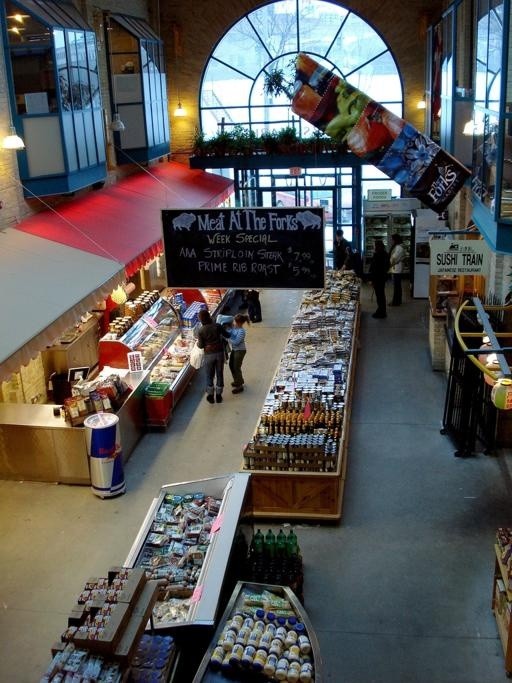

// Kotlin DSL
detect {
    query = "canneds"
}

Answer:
[109,288,159,338]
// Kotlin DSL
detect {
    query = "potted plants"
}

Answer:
[189,126,348,156]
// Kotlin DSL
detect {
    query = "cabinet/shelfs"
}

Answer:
[98,296,183,369]
[51,315,99,379]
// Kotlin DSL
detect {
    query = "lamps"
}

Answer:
[108,28,126,131]
[417,94,426,109]
[173,88,187,117]
[0,29,27,150]
[6,8,32,31]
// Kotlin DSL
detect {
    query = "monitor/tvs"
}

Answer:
[68,367,90,383]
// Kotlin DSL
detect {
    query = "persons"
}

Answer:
[246,290,262,323]
[369,237,390,319]
[333,230,352,272]
[196,310,231,403]
[222,314,247,392]
[386,233,406,306]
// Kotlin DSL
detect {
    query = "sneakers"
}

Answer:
[372,312,387,320]
[207,395,215,403]
[231,381,243,394]
[216,394,223,403]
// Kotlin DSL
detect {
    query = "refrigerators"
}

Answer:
[360,197,416,280]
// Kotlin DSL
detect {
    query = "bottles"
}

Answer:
[211,610,312,683]
[258,408,344,433]
[247,526,300,579]
[134,288,159,312]
[109,314,133,336]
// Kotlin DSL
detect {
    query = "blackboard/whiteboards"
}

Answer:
[161,207,326,290]
